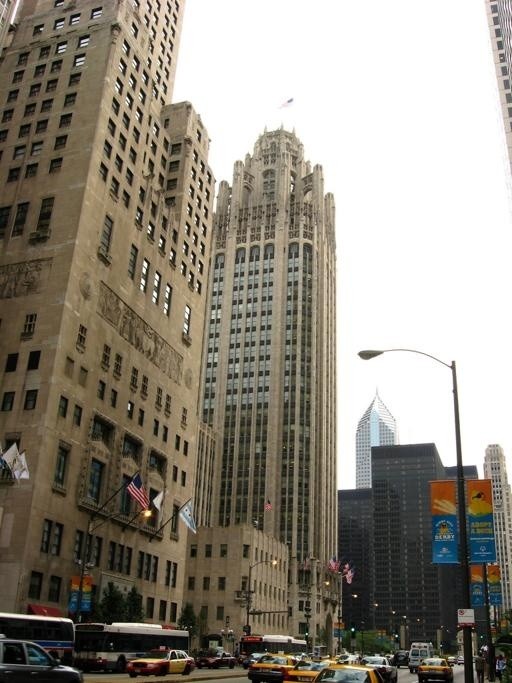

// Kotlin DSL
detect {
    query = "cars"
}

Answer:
[242,641,464,683]
[196,646,237,668]
[0,634,83,682]
[127,645,196,677]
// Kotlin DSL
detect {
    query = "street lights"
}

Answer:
[338,594,358,651]
[74,510,152,620]
[246,560,277,633]
[361,603,419,656]
[306,582,329,639]
[358,349,475,682]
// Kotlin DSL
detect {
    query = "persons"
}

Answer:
[233,648,240,665]
[473,652,505,683]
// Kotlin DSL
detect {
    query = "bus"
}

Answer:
[0,612,75,667]
[74,622,189,673]
[236,634,307,663]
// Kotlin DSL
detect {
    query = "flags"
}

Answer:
[3,444,26,483]
[329,556,354,585]
[303,554,311,570]
[126,473,150,510]
[153,487,170,529]
[11,451,30,480]
[179,499,198,535]
[264,498,273,512]
